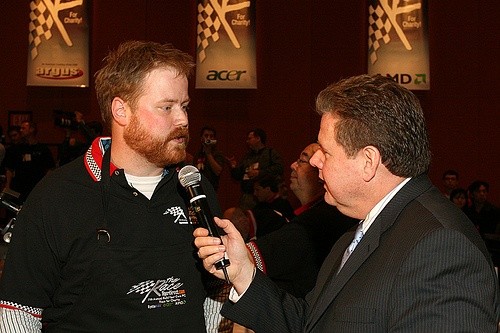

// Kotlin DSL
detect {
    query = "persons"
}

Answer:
[227,128,287,201]
[0,119,56,201]
[244,140,361,302]
[194,129,224,195]
[193,72,500,333]
[0,39,227,333]
[248,171,296,240]
[72,112,105,159]
[222,204,254,243]
[443,164,500,274]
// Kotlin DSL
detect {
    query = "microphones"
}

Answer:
[178,165,230,284]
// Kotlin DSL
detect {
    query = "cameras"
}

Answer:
[205,138,217,145]
[55,117,86,130]
